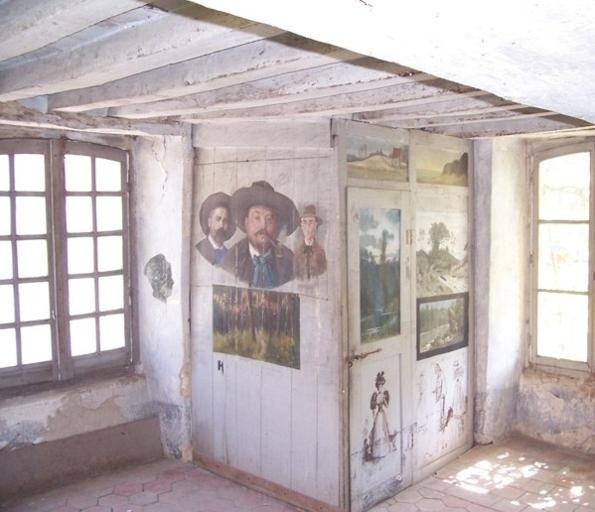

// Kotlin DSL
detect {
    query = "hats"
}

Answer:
[199,179,323,243]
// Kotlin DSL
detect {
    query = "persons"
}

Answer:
[194,191,237,264]
[294,203,326,280]
[370,372,395,460]
[218,180,301,289]
